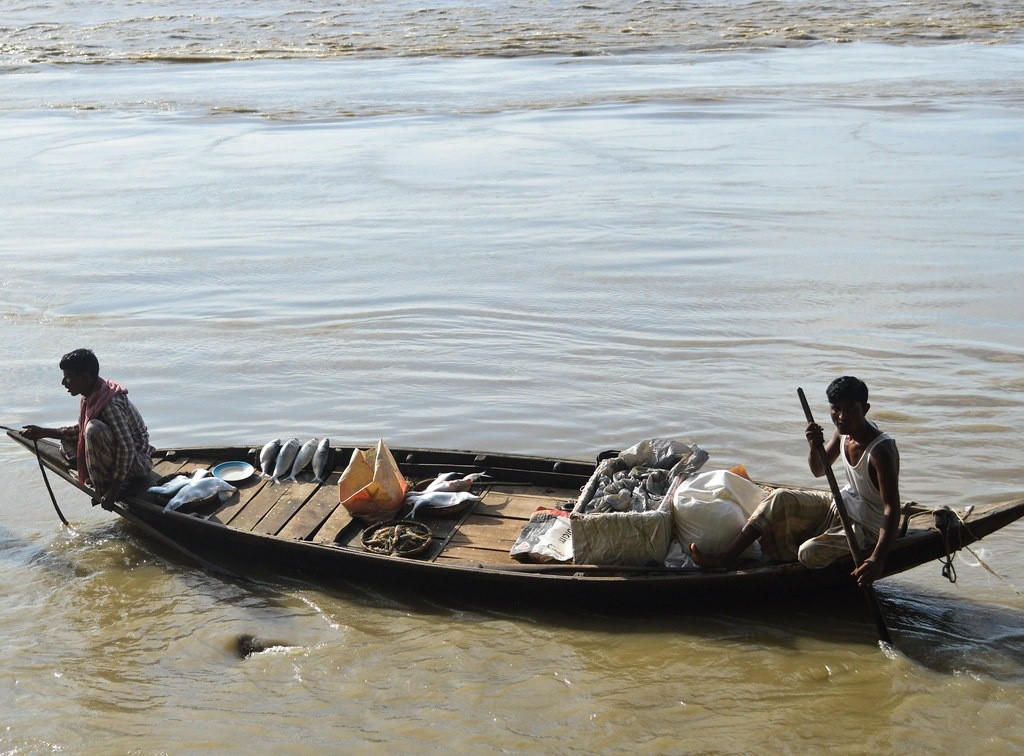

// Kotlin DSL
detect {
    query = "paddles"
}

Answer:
[796,384,896,652]
[40,460,229,574]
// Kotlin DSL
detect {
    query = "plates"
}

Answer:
[211,461,255,481]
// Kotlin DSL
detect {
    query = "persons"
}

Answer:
[18,348,156,512]
[689,375,901,589]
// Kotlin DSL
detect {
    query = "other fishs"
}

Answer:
[403,471,493,519]
[148,470,239,514]
[258,437,330,483]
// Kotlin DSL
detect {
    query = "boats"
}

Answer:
[1,424,1024,613]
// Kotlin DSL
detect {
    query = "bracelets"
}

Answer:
[864,559,875,564]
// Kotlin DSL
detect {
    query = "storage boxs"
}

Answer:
[570,458,690,568]
